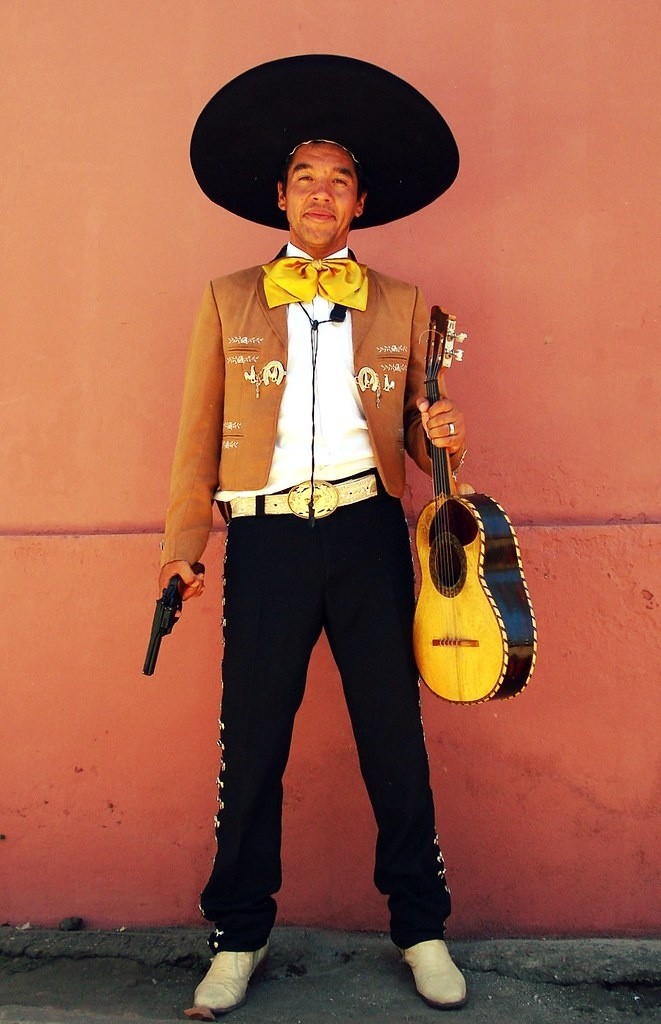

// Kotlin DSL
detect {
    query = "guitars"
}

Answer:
[412,306,539,707]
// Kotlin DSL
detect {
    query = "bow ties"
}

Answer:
[261,257,368,312]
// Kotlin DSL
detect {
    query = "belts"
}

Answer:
[230,475,378,519]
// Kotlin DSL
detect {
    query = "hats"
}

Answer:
[191,55,460,231]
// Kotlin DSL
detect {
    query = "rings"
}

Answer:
[448,423,455,435]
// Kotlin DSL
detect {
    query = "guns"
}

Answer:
[140,562,207,676]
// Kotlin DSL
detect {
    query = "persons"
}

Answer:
[154,54,471,1009]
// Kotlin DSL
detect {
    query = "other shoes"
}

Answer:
[397,940,467,1009]
[194,942,269,1012]
